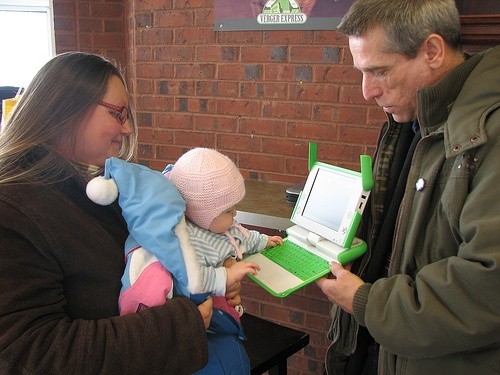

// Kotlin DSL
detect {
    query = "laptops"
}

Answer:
[237,142,373,297]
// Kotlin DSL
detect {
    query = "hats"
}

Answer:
[167,147,246,231]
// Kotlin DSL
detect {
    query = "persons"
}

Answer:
[314,1,499,374]
[0,51,242,375]
[102,147,284,375]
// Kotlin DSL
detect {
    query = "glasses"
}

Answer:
[96,101,129,125]
